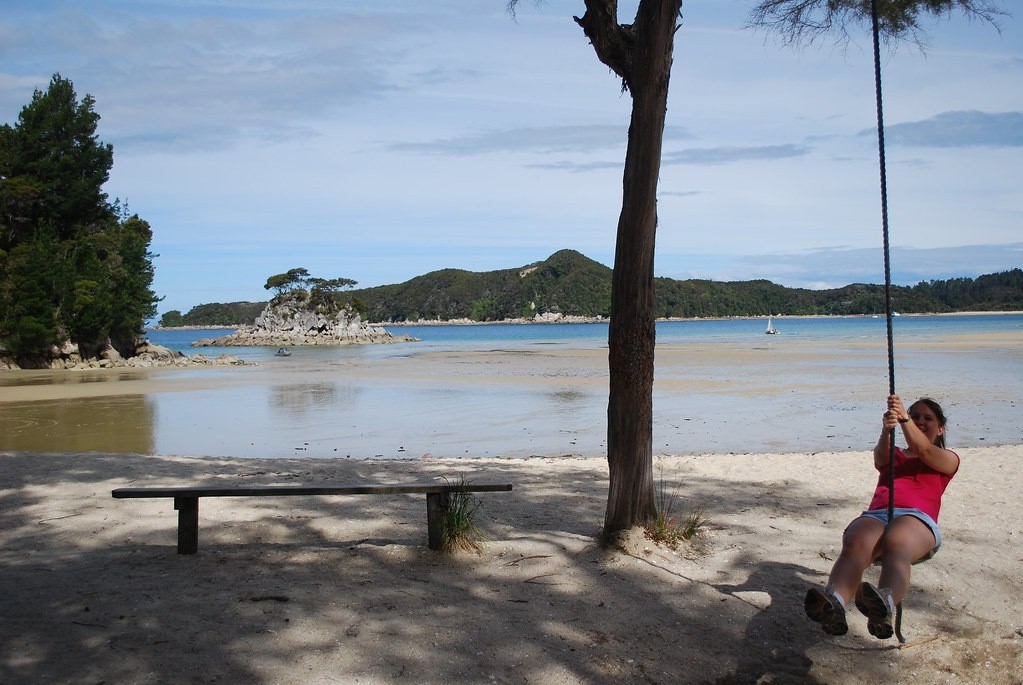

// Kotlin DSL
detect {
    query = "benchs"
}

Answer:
[113,481,512,556]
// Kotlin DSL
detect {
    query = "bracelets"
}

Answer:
[898,415,911,423]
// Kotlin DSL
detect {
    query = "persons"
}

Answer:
[804,395,960,640]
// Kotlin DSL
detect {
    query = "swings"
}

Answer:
[870,0,936,567]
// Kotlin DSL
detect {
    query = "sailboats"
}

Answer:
[764,308,778,334]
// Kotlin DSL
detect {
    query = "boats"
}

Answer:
[274,347,292,357]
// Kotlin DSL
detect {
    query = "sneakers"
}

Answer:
[804,588,848,637]
[854,581,894,640]
